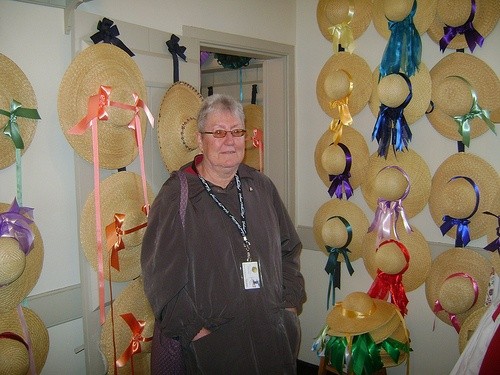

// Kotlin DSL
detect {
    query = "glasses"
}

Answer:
[201,129,246,138]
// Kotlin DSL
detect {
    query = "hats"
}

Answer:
[313,198,370,311]
[425,247,497,335]
[57,44,156,327]
[360,145,432,251]
[425,52,500,149]
[79,170,156,283]
[98,276,156,375]
[0,196,44,375]
[311,292,410,375]
[458,306,488,354]
[157,80,205,174]
[361,223,432,318]
[316,0,373,56]
[368,57,432,161]
[373,0,438,78]
[313,125,370,201]
[426,0,500,55]
[0,305,50,375]
[242,104,264,174]
[0,53,42,208]
[482,192,500,256]
[428,152,500,249]
[316,51,374,146]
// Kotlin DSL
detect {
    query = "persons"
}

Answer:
[141,93,306,375]
